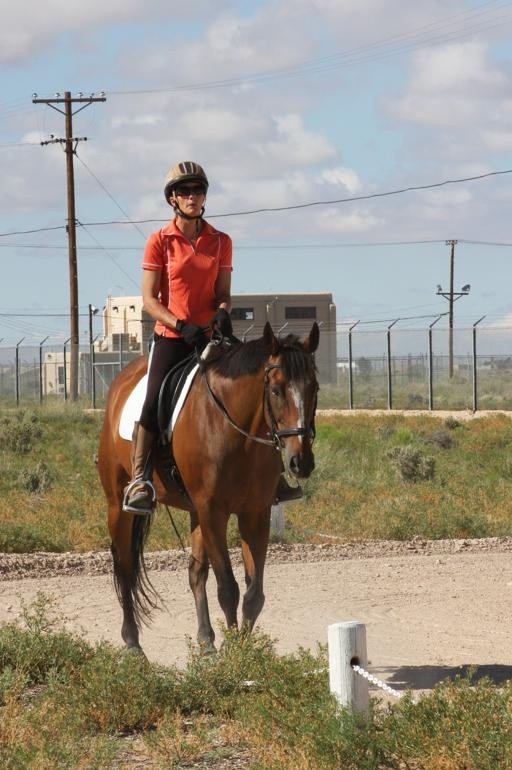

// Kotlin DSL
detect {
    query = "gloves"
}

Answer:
[175,319,206,348]
[208,307,234,337]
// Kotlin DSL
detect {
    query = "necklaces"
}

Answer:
[189,221,199,242]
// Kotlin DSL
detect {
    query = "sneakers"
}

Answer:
[276,475,304,506]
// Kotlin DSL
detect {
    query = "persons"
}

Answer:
[126,162,302,512]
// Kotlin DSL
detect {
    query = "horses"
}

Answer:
[97,316,322,671]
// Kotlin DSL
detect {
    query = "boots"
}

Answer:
[121,417,160,515]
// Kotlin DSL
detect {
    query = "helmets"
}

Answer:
[163,160,210,193]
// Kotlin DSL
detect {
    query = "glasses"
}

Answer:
[173,185,208,195]
